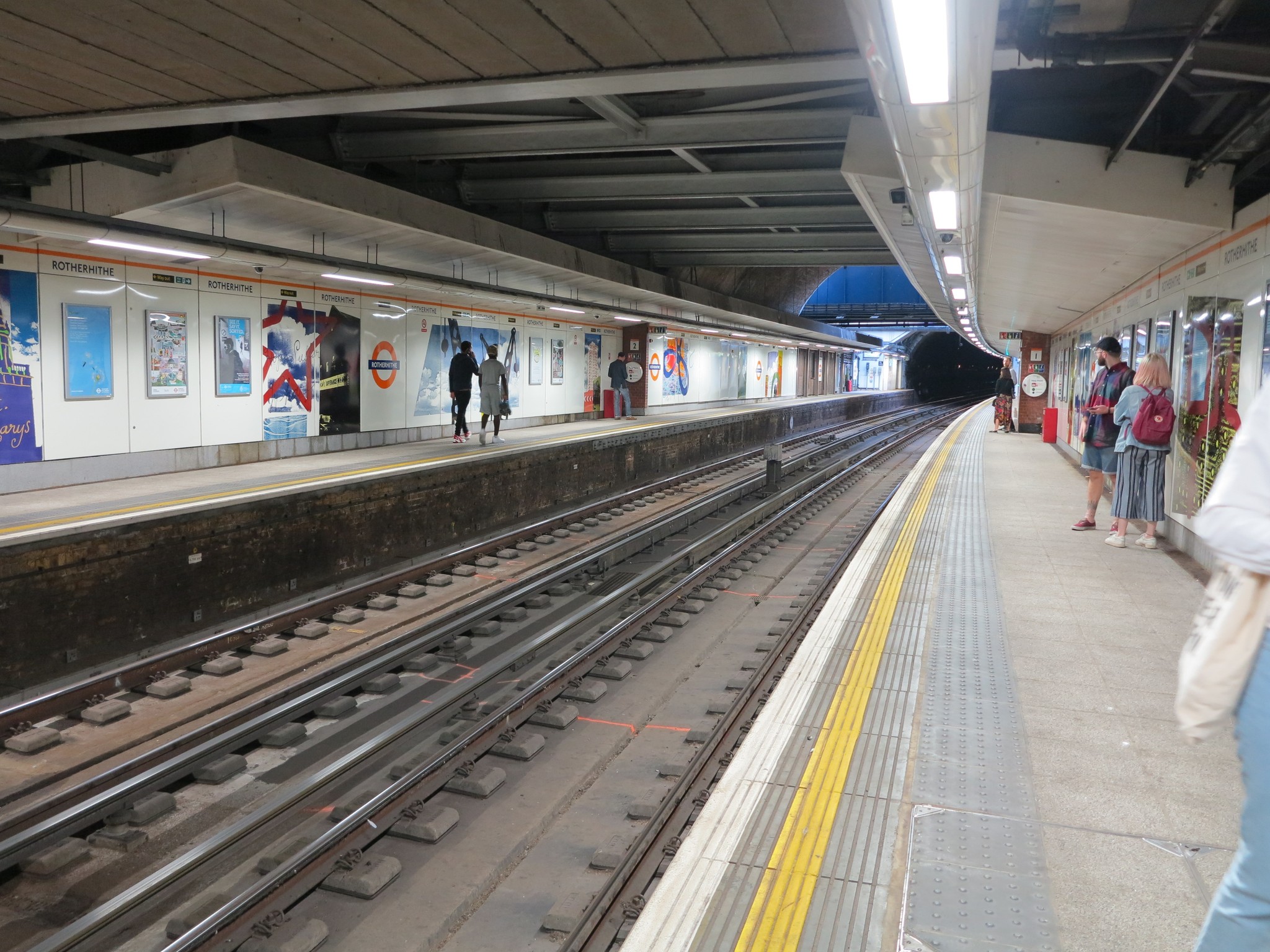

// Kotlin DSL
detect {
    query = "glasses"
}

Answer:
[1094,350,1103,354]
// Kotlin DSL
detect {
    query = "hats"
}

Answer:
[487,345,498,357]
[1092,337,1121,353]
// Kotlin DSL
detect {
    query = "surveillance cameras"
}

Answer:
[936,231,958,244]
[252,265,267,274]
[593,314,600,319]
[727,334,732,337]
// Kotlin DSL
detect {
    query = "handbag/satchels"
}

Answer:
[992,398,997,407]
[1175,558,1270,746]
[499,400,511,416]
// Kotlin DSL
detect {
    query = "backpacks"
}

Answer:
[1124,384,1177,446]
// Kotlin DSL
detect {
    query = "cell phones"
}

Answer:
[1086,407,1095,410]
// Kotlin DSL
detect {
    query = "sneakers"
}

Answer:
[1105,534,1125,548]
[1004,431,1010,434]
[479,428,486,445]
[998,427,1006,431]
[1135,533,1156,549]
[1109,524,1118,535]
[453,434,466,443]
[464,431,472,440]
[989,430,998,434]
[492,436,505,443]
[1010,428,1016,432]
[1071,520,1096,530]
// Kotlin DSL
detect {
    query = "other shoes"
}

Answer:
[626,416,637,420]
[615,418,621,420]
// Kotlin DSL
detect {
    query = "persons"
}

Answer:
[608,352,637,420]
[1105,352,1175,549]
[223,338,245,384]
[449,341,479,443]
[1070,336,1136,535]
[989,356,1017,434]
[240,341,243,355]
[1175,370,1270,952]
[477,343,509,444]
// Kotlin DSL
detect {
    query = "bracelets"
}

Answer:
[1108,407,1110,414]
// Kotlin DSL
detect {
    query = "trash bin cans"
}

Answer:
[604,389,622,418]
[846,380,852,391]
[1042,407,1058,443]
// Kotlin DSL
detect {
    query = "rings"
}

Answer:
[1094,412,1095,415]
